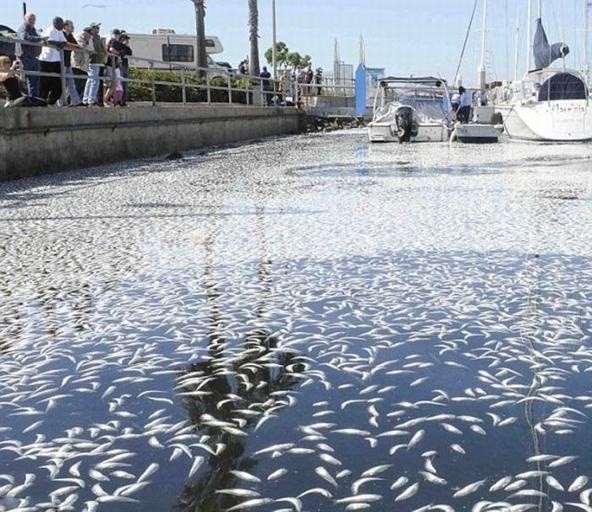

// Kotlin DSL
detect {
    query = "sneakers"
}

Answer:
[76,101,128,108]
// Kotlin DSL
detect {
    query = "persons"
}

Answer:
[456,86,471,124]
[297,66,322,95]
[0,13,132,108]
[260,66,271,85]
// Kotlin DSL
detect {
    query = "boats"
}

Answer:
[367,0,592,143]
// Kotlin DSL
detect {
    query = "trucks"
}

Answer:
[106,28,233,88]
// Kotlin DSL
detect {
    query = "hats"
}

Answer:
[90,21,102,28]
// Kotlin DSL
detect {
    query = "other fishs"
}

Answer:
[1,131,591,511]
